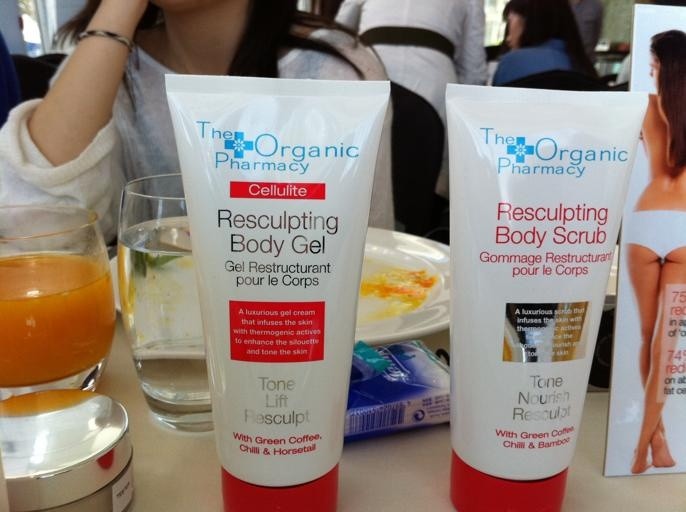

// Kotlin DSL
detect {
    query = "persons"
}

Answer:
[625,27,686,474]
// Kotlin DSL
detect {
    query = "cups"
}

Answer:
[116,173,217,434]
[0,205,116,404]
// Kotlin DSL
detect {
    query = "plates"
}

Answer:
[110,226,450,346]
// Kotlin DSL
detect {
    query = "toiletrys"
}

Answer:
[444,83,651,512]
[163,74,392,512]
